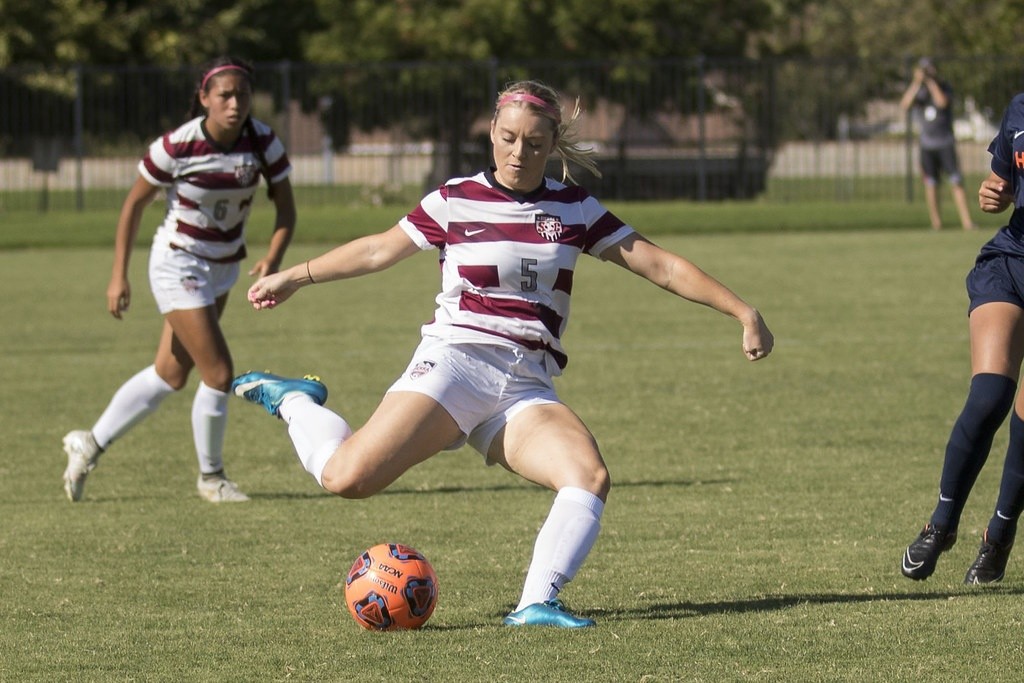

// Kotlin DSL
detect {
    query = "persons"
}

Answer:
[62,57,297,504]
[230,81,775,629]
[902,94,1024,584]
[902,57,978,231]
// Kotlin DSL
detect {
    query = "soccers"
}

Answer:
[344,543,438,630]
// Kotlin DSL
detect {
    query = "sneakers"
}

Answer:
[963,527,1017,587]
[197,474,251,504]
[504,598,596,628]
[902,524,957,581]
[232,369,328,419]
[62,430,105,502]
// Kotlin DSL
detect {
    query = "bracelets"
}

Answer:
[307,260,315,284]
